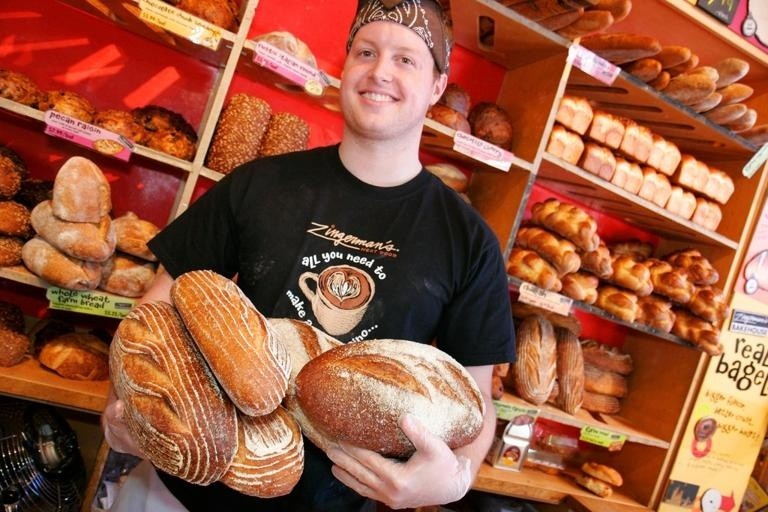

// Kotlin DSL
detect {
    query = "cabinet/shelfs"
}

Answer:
[499,2,766,511]
[0,0,257,511]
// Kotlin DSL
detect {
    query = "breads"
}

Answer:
[1,147,163,298]
[545,94,735,232]
[108,270,487,499]
[505,197,730,356]
[497,1,767,146]
[490,299,635,416]
[171,1,245,34]
[253,30,318,95]
[256,112,312,160]
[205,93,272,176]
[426,82,513,151]
[424,162,472,205]
[522,417,623,499]
[2,69,199,162]
[0,309,113,382]
